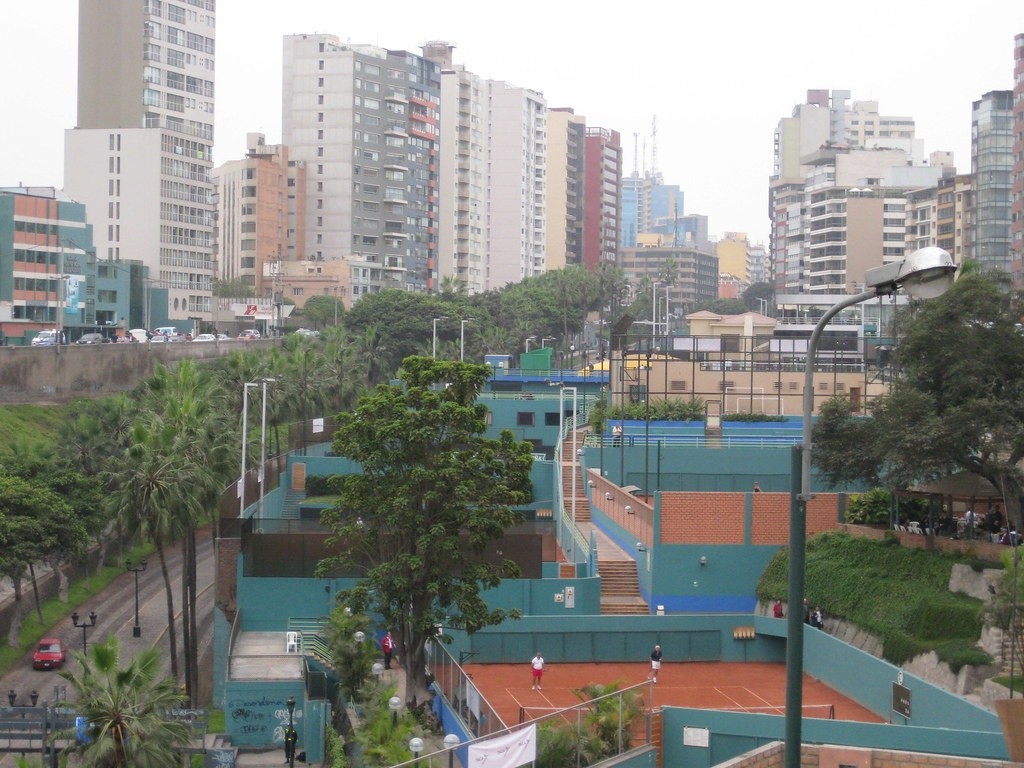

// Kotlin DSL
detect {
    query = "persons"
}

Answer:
[155,328,193,342]
[531,652,544,690]
[283,723,298,764]
[753,481,763,492]
[223,326,230,336]
[109,330,137,342]
[903,501,1023,547]
[803,597,810,624]
[383,630,396,671]
[773,599,785,618]
[810,604,824,630]
[611,422,622,447]
[647,645,663,683]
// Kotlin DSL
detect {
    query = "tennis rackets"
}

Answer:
[530,665,554,672]
[647,665,654,680]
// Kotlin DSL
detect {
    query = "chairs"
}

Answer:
[286,632,298,652]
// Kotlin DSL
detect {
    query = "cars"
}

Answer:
[293,328,320,337]
[32,637,67,669]
[149,334,235,342]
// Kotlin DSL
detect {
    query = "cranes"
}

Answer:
[632,112,658,177]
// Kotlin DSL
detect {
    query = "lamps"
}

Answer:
[587,479,596,489]
[636,541,646,552]
[624,506,635,516]
[700,556,708,566]
[605,492,612,502]
[577,448,584,456]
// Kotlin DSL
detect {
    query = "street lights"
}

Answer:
[432,316,449,390]
[304,348,312,455]
[549,381,563,501]
[653,283,662,348]
[287,695,297,768]
[460,316,475,361]
[563,386,576,526]
[258,378,275,527]
[525,336,538,353]
[784,246,959,768]
[125,558,148,637]
[237,382,258,518]
[71,611,97,656]
[541,336,556,349]
[756,297,768,317]
[666,285,674,335]
[8,689,39,756]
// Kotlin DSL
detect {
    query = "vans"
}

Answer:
[31,330,66,346]
[238,329,260,340]
[154,327,177,336]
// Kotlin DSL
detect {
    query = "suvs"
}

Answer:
[75,333,103,344]
